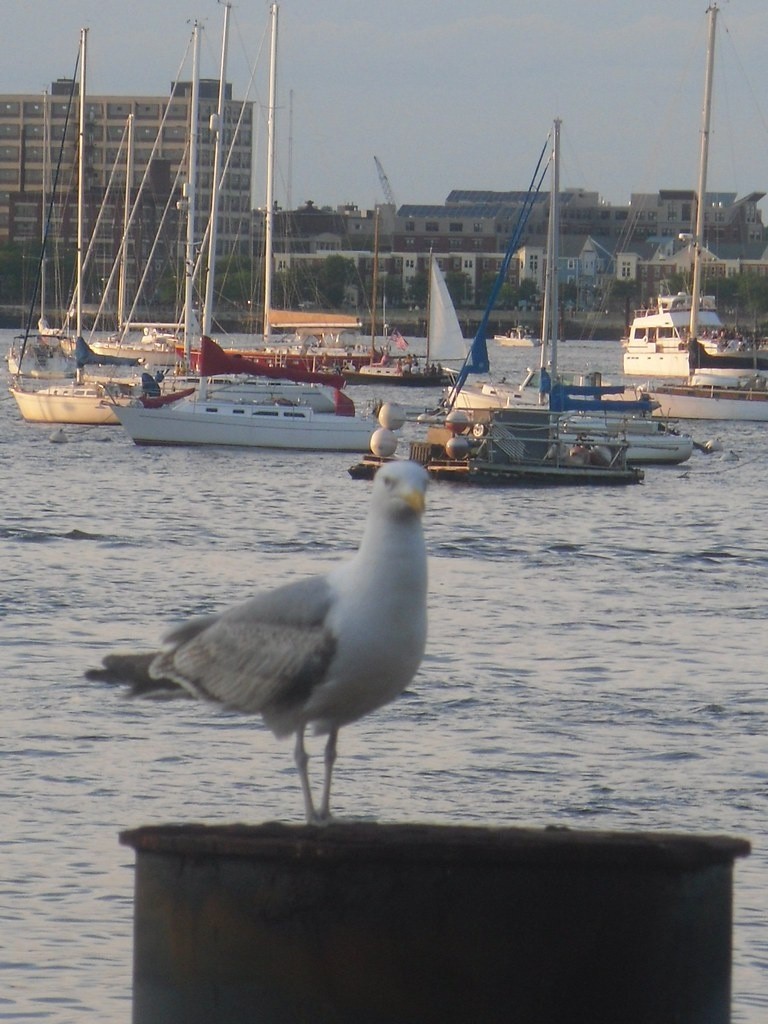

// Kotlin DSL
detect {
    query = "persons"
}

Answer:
[396,353,445,376]
[700,327,766,353]
[544,434,617,468]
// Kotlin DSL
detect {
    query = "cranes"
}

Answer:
[373,156,395,204]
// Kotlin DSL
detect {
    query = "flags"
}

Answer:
[390,328,409,351]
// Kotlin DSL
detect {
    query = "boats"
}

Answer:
[348,402,646,488]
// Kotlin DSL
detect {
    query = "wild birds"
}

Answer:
[82,459,432,828]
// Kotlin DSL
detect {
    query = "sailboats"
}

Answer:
[0,0,768,466]
